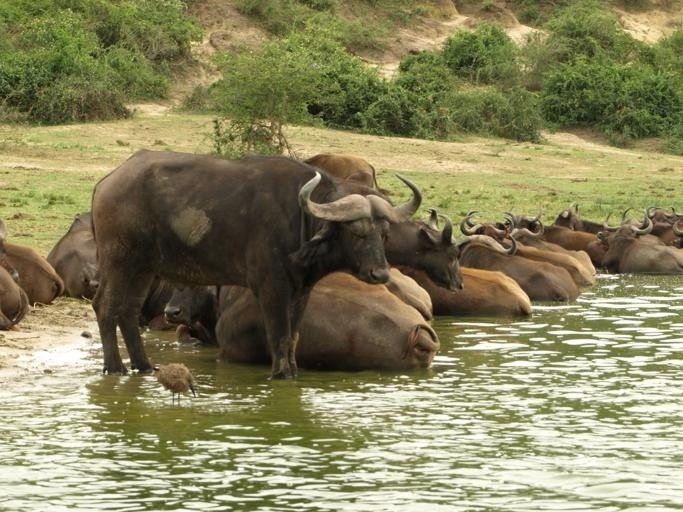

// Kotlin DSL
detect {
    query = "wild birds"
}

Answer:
[153,361,199,407]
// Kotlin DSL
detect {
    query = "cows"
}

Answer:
[0,265,29,330]
[0,219,65,305]
[447,201,683,320]
[90,153,463,379]
[47,211,98,299]
